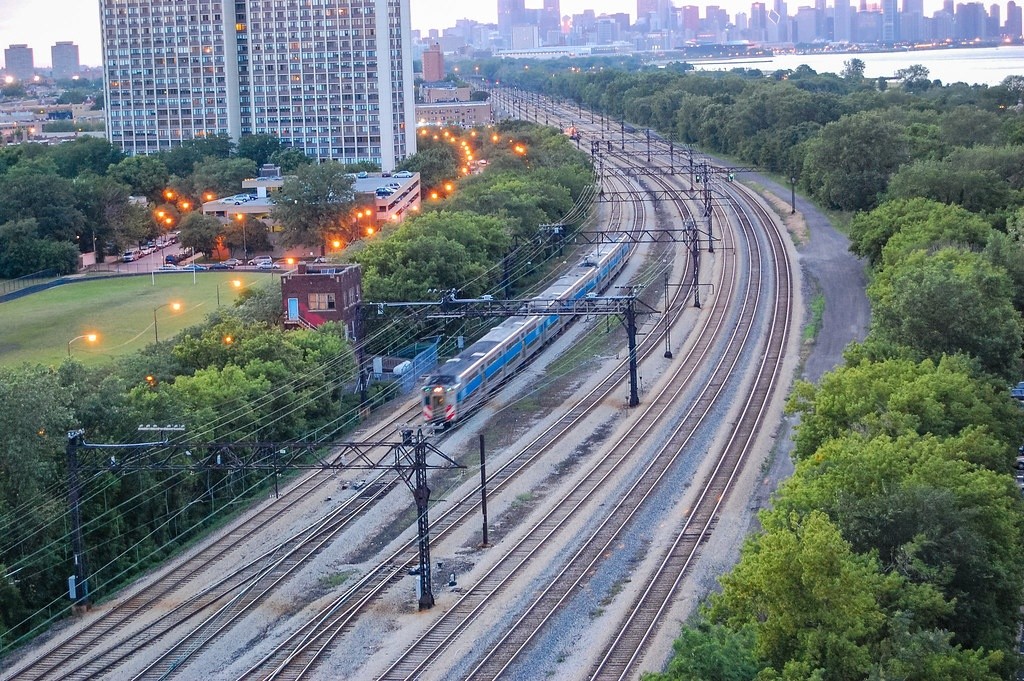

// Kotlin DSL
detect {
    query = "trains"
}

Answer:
[421,228,635,429]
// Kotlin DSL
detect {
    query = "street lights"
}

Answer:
[238,214,248,264]
[153,302,181,343]
[159,211,171,267]
[215,280,241,306]
[67,334,97,357]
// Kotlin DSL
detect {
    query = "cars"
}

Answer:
[218,197,244,206]
[375,182,401,196]
[393,170,414,178]
[210,262,235,269]
[219,259,243,266]
[234,193,258,203]
[256,259,294,270]
[315,256,330,263]
[122,231,207,265]
[182,264,208,270]
[358,170,369,179]
[158,264,177,270]
[382,170,391,177]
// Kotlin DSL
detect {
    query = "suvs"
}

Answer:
[247,254,274,266]
